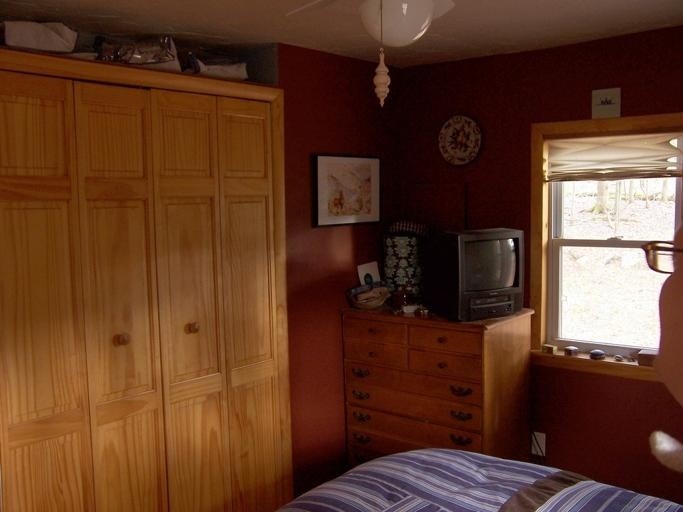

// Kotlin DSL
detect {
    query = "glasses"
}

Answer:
[639,241,683,274]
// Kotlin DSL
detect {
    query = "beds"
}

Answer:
[278,447,683,512]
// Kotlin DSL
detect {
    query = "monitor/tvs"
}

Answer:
[425,225,526,321]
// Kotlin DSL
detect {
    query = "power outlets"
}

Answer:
[532,432,546,457]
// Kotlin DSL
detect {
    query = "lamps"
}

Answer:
[373,0,391,108]
[360,0,434,47]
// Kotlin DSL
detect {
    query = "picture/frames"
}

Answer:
[438,115,483,167]
[315,154,383,227]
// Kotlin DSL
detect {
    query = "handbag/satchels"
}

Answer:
[99,33,181,71]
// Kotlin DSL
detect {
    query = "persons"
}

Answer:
[640,225,683,404]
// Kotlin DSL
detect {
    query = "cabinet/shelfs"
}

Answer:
[334,307,536,469]
[1,48,293,512]
